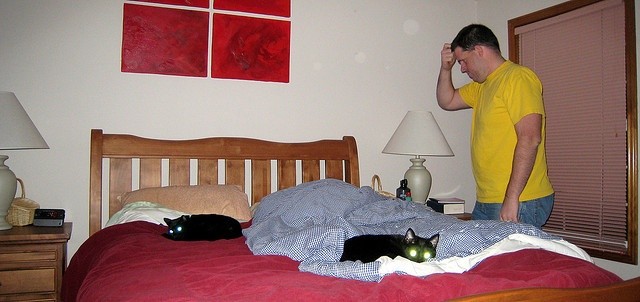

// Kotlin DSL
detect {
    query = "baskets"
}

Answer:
[4,178,40,227]
[372,174,395,198]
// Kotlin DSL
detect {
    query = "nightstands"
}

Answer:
[0,224,74,301]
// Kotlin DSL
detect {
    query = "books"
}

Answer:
[429,198,465,204]
[426,200,466,215]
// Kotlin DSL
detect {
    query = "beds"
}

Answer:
[61,130,640,302]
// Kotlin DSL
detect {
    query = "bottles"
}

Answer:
[396,179,411,200]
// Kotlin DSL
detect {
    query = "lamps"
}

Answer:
[0,92,50,232]
[381,110,455,205]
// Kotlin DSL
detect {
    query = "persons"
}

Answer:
[436,24,554,228]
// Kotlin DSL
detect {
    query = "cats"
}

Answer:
[339,227,440,263]
[163,213,244,241]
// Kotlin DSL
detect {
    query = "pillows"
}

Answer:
[116,185,252,223]
